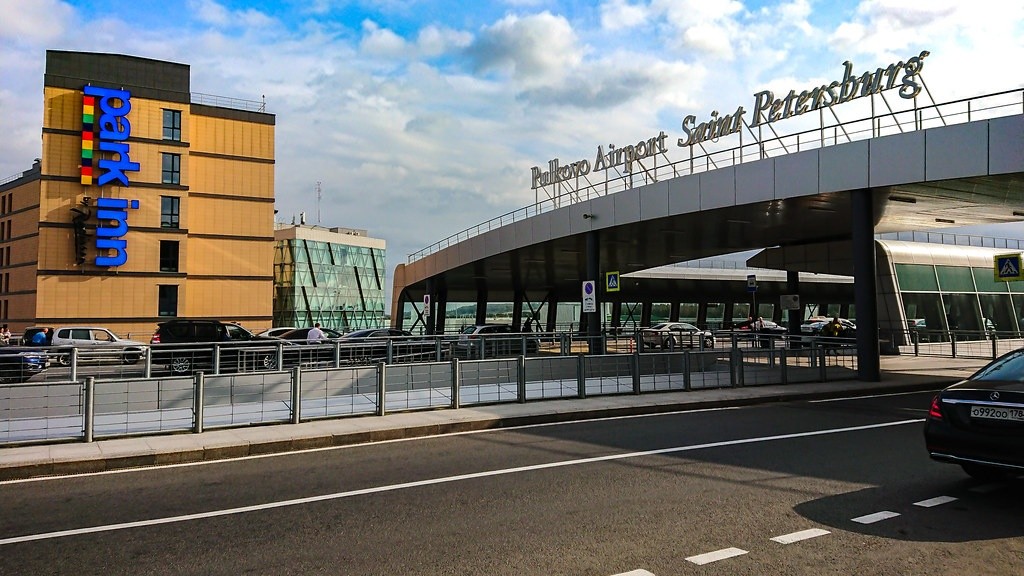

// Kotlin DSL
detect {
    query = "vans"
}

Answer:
[19,326,56,360]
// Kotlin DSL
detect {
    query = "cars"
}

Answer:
[924,347,1024,486]
[257,326,298,337]
[278,328,342,363]
[906,317,929,341]
[0,340,48,384]
[457,322,540,356]
[799,317,858,346]
[635,322,714,350]
[325,327,438,365]
[731,319,789,342]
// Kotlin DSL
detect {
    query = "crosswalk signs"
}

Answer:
[606,271,620,291]
[994,255,1024,282]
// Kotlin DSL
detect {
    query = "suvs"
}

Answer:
[144,318,292,375]
[48,327,147,366]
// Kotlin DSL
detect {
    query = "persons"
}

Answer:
[524,317,532,332]
[749,317,765,331]
[32,328,48,346]
[570,323,573,332]
[0,328,11,345]
[307,323,329,345]
